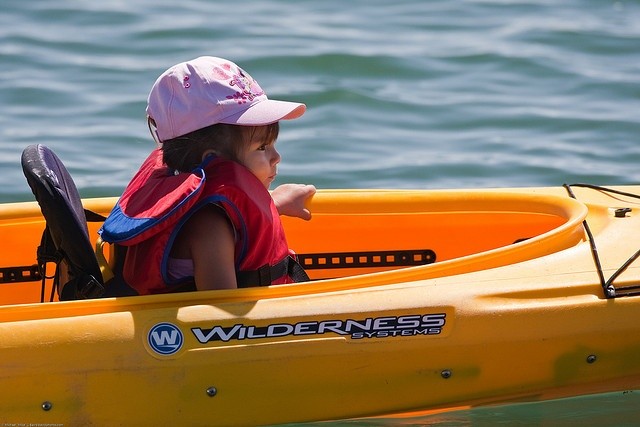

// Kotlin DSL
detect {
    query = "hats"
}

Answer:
[146,56,306,143]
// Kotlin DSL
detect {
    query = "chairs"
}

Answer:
[21,144,106,302]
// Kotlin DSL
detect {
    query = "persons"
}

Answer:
[94,56,317,299]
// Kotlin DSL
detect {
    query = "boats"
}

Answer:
[0,184,640,426]
